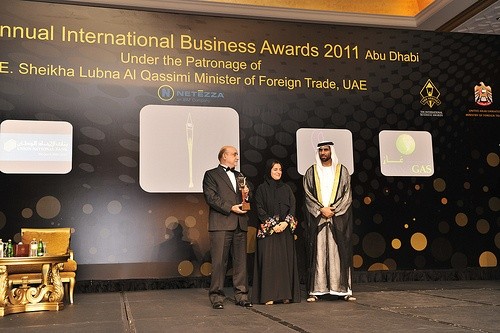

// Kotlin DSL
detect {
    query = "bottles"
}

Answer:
[0,238,46,259]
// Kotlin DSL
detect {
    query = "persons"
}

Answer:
[203,146,254,309]
[252,159,300,305]
[303,137,356,302]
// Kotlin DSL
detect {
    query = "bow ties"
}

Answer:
[222,166,235,172]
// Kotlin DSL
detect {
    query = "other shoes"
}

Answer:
[307,295,319,302]
[344,295,356,301]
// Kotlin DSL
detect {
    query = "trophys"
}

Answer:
[237,173,250,210]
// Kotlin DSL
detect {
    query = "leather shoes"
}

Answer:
[211,301,223,309]
[240,300,253,306]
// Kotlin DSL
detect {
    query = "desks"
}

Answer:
[0,252,70,317]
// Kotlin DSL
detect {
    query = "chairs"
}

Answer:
[8,227,76,304]
